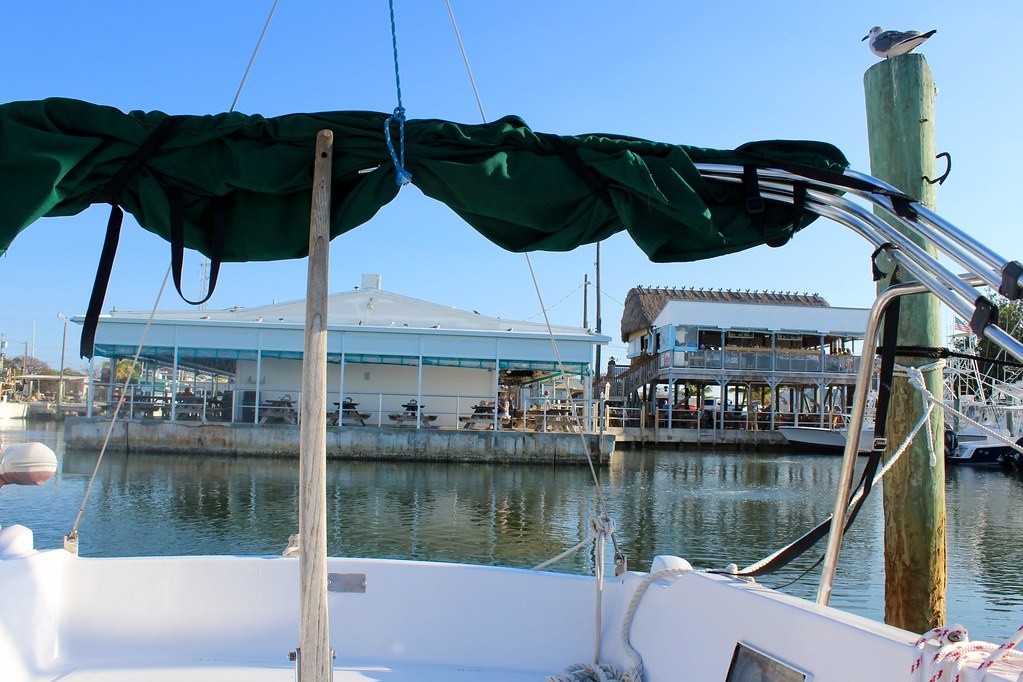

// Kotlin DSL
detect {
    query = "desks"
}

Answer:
[258,400,300,425]
[510,409,542,431]
[327,402,372,426]
[458,405,503,430]
[388,405,438,429]
[176,396,214,422]
[528,409,576,434]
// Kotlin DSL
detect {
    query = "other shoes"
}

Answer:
[485,428,492,430]
[492,428,499,430]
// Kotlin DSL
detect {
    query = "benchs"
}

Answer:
[99,396,577,434]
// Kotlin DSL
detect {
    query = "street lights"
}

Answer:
[57,312,68,418]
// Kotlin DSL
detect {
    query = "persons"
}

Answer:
[541,391,551,405]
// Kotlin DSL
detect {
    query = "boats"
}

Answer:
[777,386,881,453]
[943,318,1023,471]
